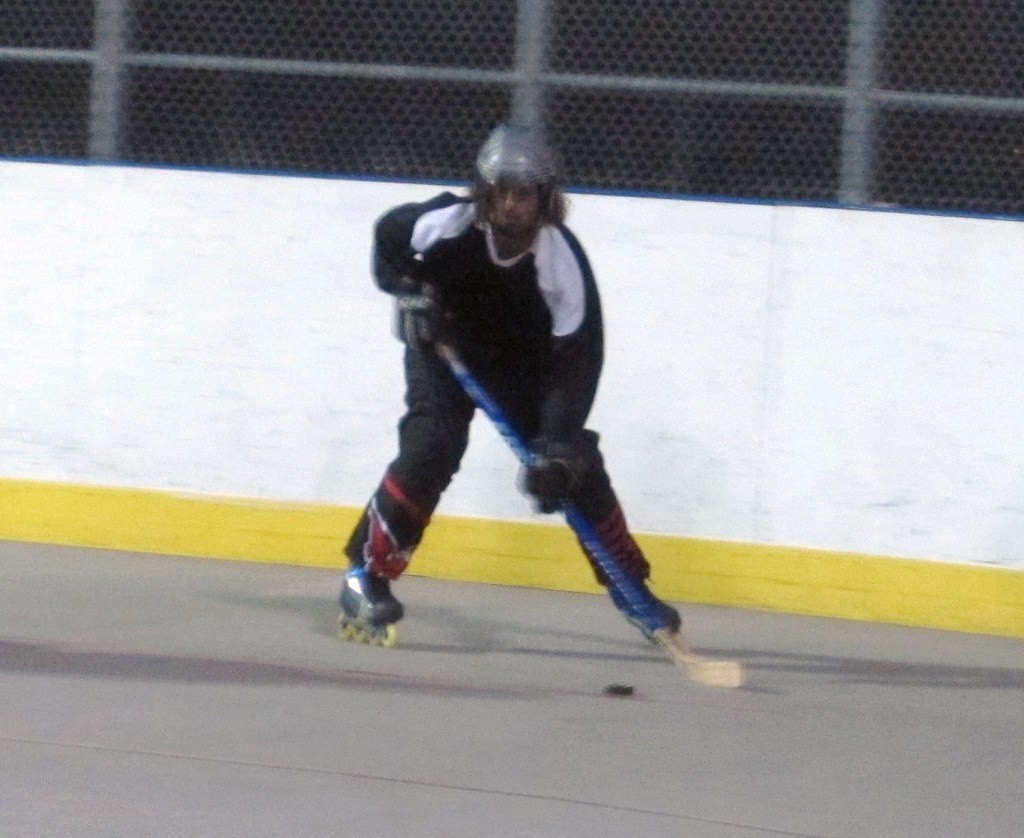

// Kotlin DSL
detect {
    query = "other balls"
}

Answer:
[603,683,633,695]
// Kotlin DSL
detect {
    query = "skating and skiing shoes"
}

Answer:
[337,572,403,649]
[608,579,680,644]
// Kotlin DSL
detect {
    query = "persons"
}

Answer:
[339,125,681,656]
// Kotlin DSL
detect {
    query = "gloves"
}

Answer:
[394,293,441,351]
[518,446,580,516]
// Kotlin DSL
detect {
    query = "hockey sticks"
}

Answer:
[435,338,744,687]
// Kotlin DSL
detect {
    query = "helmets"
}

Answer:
[475,123,562,187]
